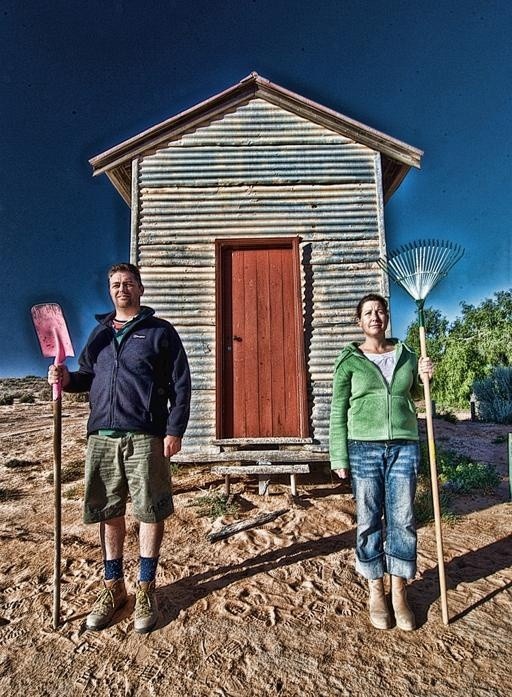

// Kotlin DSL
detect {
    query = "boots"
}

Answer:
[367,577,392,630]
[133,578,159,633]
[84,576,128,631]
[390,575,417,632]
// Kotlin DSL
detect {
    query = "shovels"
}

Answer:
[31,302,76,631]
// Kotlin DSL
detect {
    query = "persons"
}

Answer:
[329,294,434,630]
[47,262,191,633]
